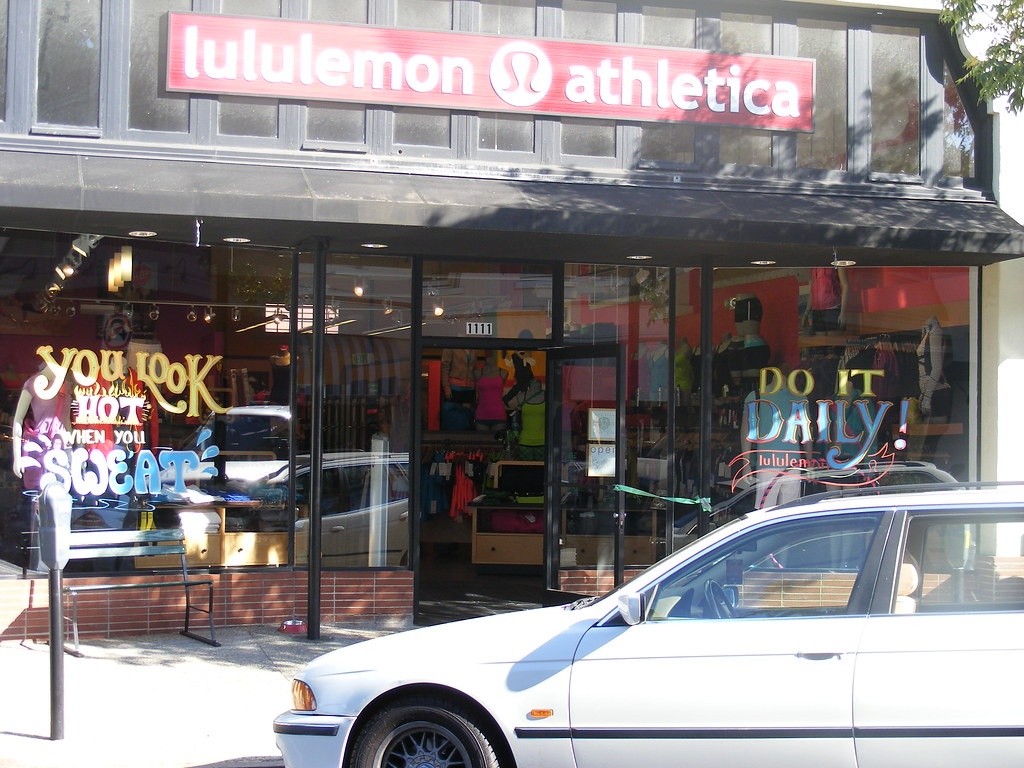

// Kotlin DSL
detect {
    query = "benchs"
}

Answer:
[60,525,221,659]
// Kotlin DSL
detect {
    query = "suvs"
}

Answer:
[671,461,973,570]
[270,480,1023,768]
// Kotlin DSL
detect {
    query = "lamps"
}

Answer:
[32,235,487,337]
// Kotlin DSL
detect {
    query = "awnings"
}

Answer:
[0,152,1023,266]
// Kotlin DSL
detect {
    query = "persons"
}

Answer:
[916,315,951,458]
[800,268,848,336]
[123,287,161,378]
[441,333,478,428]
[628,332,771,400]
[79,357,160,482]
[816,381,877,569]
[12,365,75,490]
[269,346,298,404]
[474,357,508,430]
[510,381,545,461]
[739,362,816,568]
[735,294,762,333]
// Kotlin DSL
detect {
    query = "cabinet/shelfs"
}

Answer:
[468,500,657,577]
[134,500,298,570]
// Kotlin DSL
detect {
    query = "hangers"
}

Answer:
[805,333,918,361]
[420,439,516,463]
[665,425,733,448]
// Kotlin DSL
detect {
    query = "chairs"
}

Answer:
[893,548,921,613]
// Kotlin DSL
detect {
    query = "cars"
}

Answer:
[255,452,411,567]
[146,407,292,486]
[27,496,127,572]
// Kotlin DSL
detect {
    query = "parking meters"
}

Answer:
[42,481,72,742]
